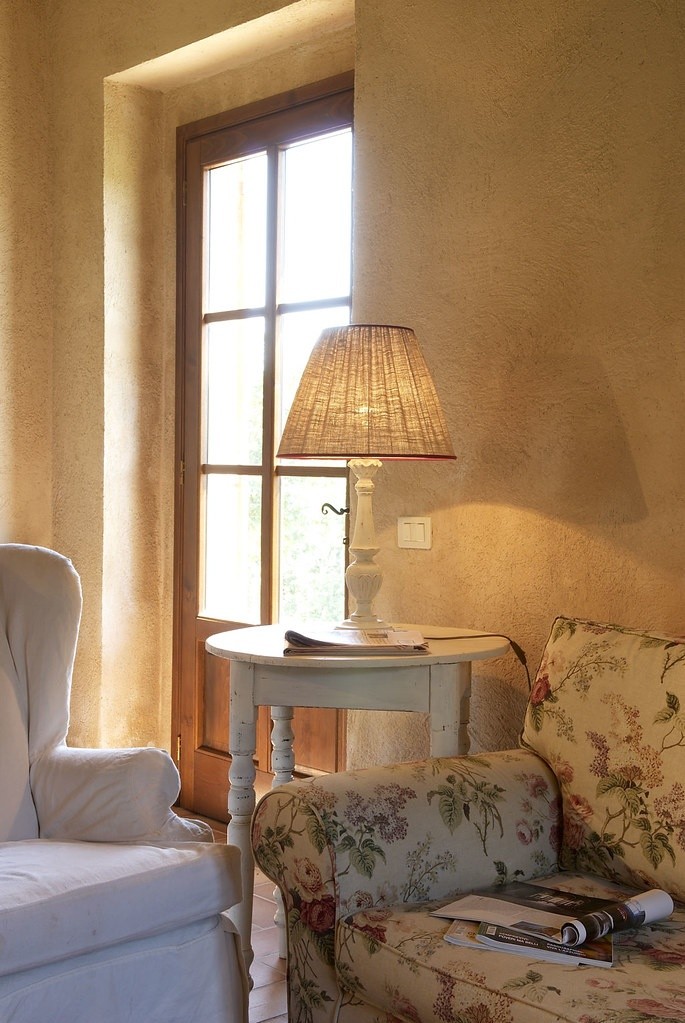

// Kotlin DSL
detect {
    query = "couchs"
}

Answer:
[0,544,258,1023]
[241,616,685,1023]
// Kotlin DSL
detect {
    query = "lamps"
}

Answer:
[277,324,457,634]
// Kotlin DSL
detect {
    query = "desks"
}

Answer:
[205,625,510,993]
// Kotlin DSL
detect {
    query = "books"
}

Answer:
[429,880,674,969]
